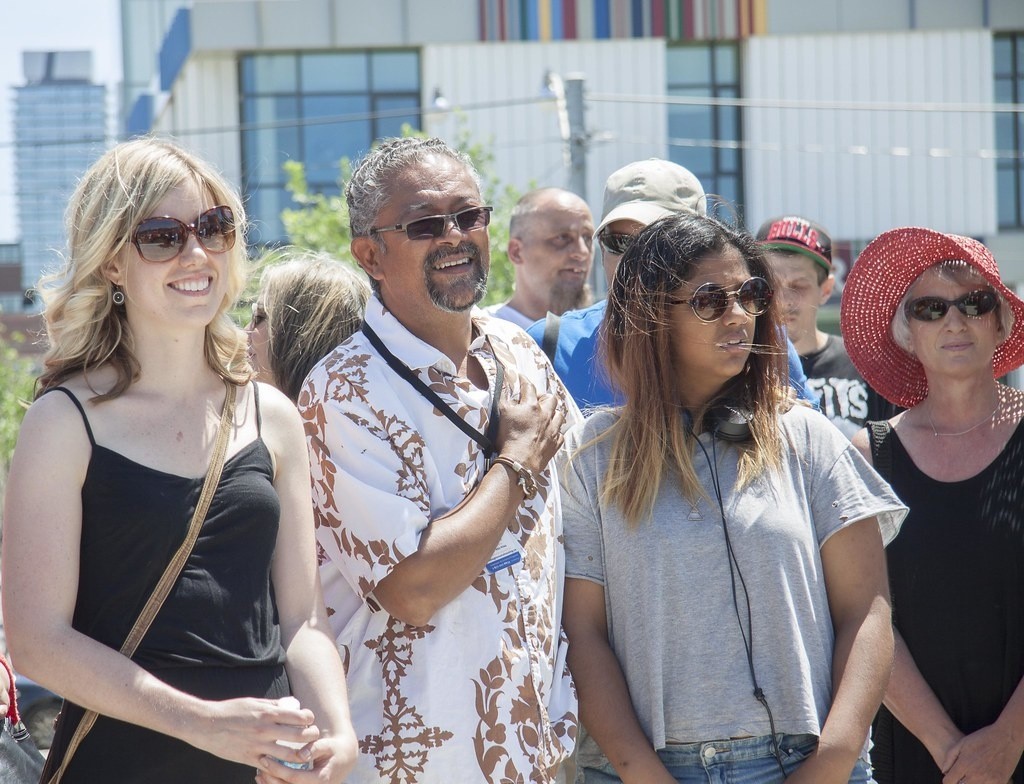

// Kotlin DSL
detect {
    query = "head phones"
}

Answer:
[679,397,757,443]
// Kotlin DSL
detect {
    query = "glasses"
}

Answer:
[907,289,1001,323]
[668,277,775,323]
[249,302,268,329]
[598,232,639,255]
[116,205,237,263]
[372,203,493,241]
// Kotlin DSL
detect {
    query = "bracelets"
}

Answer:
[491,457,538,499]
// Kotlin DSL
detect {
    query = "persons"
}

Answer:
[551,215,910,784]
[527,158,820,421]
[755,214,910,442]
[840,227,1024,784]
[480,188,595,331]
[0,140,359,784]
[296,137,586,784]
[245,257,366,405]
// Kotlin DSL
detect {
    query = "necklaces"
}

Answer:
[663,465,713,521]
[923,399,1001,436]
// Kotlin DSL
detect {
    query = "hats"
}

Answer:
[750,217,835,269]
[839,226,1024,409]
[592,158,709,238]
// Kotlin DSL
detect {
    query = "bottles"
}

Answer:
[256,694,314,776]
